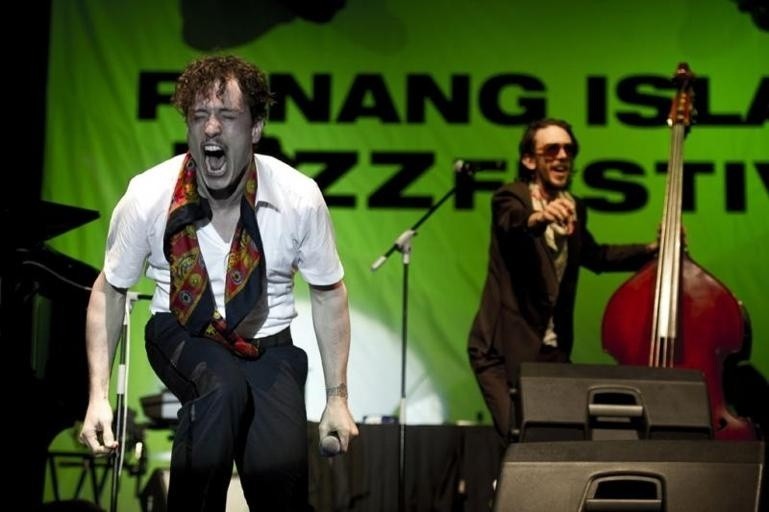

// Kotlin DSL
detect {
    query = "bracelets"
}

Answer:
[323,382,349,400]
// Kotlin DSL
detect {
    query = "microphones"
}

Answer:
[455,160,468,180]
[490,439,765,512]
[510,362,713,442]
[321,432,341,453]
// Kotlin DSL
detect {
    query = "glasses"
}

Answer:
[535,144,577,158]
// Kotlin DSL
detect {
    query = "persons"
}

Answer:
[460,117,689,442]
[73,51,361,511]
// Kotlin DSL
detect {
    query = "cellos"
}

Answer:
[602,61,767,440]
